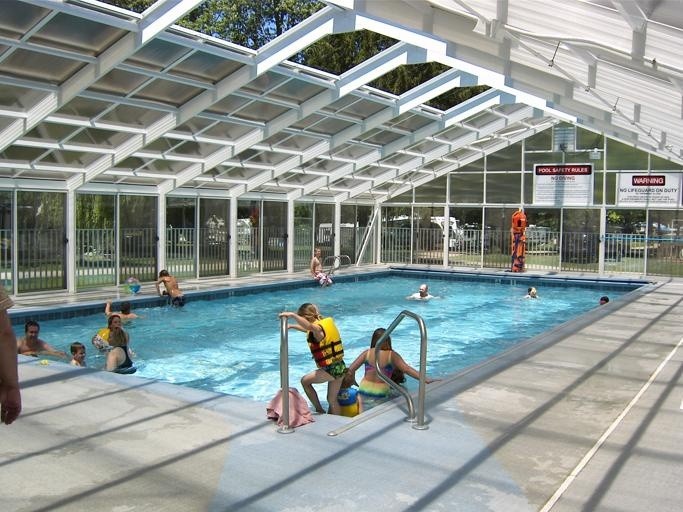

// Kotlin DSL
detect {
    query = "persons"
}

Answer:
[68,342,87,367]
[327,367,362,417]
[599,296,609,306]
[104,298,144,320]
[16,321,64,359]
[347,328,442,405]
[389,368,408,397]
[523,286,538,300]
[155,270,184,306]
[92,314,128,352]
[310,248,334,286]
[278,302,346,415]
[102,326,136,374]
[405,283,440,302]
[0,285,21,425]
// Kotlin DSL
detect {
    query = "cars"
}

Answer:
[568,217,681,261]
[77,242,115,258]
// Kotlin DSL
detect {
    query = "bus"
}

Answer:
[380,214,559,254]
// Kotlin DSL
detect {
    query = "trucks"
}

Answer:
[205,216,253,258]
[314,220,361,247]
